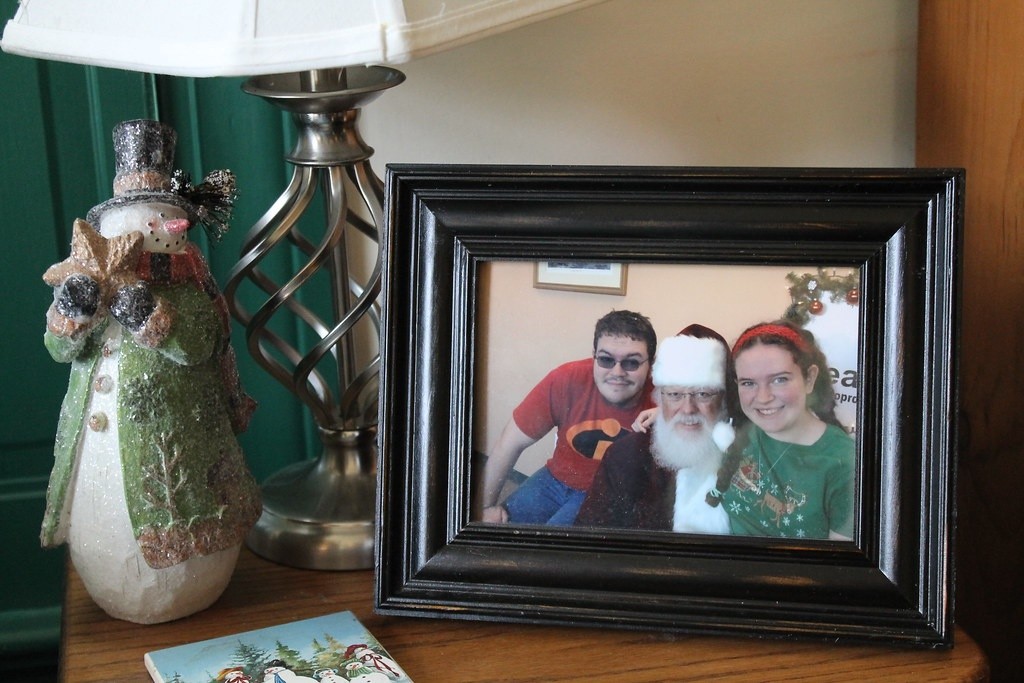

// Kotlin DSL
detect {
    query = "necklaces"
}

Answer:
[756,429,794,496]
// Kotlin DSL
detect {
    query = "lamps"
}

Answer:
[3,0,596,575]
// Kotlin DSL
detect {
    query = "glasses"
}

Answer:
[594,355,649,371]
[659,391,718,403]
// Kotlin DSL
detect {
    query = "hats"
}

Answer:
[650,324,732,389]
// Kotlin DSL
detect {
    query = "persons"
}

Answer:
[470,310,856,541]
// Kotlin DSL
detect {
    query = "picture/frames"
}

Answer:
[532,260,629,297]
[368,161,967,653]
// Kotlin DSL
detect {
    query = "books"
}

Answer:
[144,610,414,683]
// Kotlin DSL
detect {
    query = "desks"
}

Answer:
[59,545,994,683]
